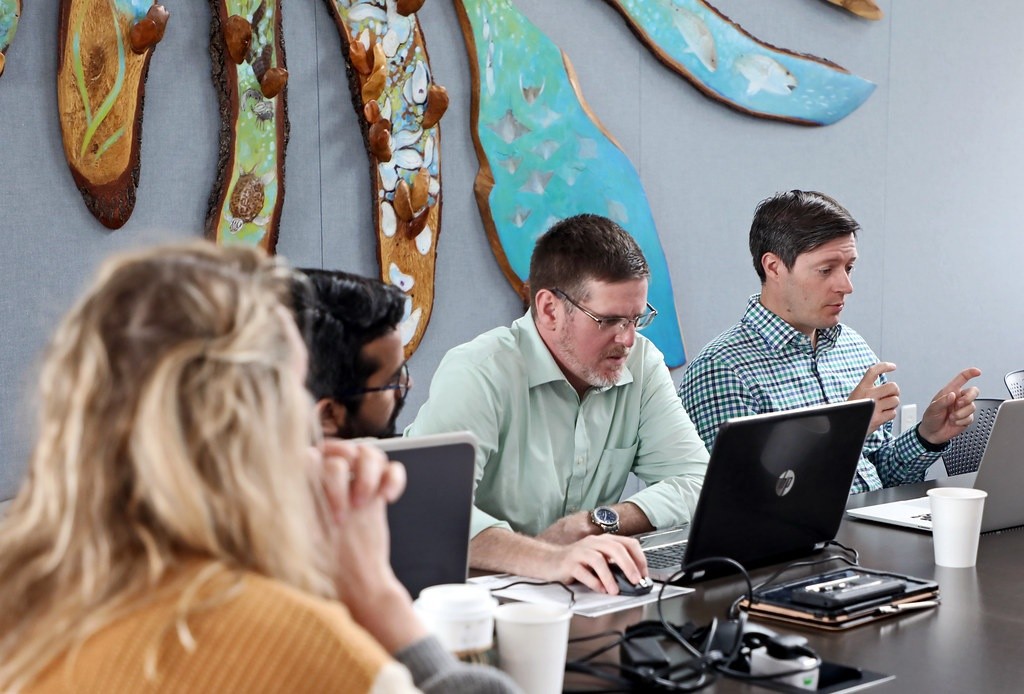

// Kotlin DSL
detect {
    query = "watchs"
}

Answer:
[590,506,619,535]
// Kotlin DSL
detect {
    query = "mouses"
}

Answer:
[588,563,654,596]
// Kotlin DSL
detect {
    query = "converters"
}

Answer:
[618,637,672,684]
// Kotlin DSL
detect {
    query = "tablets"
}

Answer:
[743,564,938,618]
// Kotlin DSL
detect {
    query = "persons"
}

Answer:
[401,214,711,597]
[677,190,982,493]
[291,268,413,438]
[0,243,522,694]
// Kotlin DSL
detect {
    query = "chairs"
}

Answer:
[1004,370,1024,400]
[942,398,1005,478]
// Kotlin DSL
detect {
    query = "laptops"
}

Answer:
[606,398,877,589]
[361,431,479,602]
[845,397,1024,534]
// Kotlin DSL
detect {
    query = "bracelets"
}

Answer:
[916,423,946,451]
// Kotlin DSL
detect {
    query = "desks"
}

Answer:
[468,470,1024,694]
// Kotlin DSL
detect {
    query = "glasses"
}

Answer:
[552,287,660,333]
[333,362,409,400]
